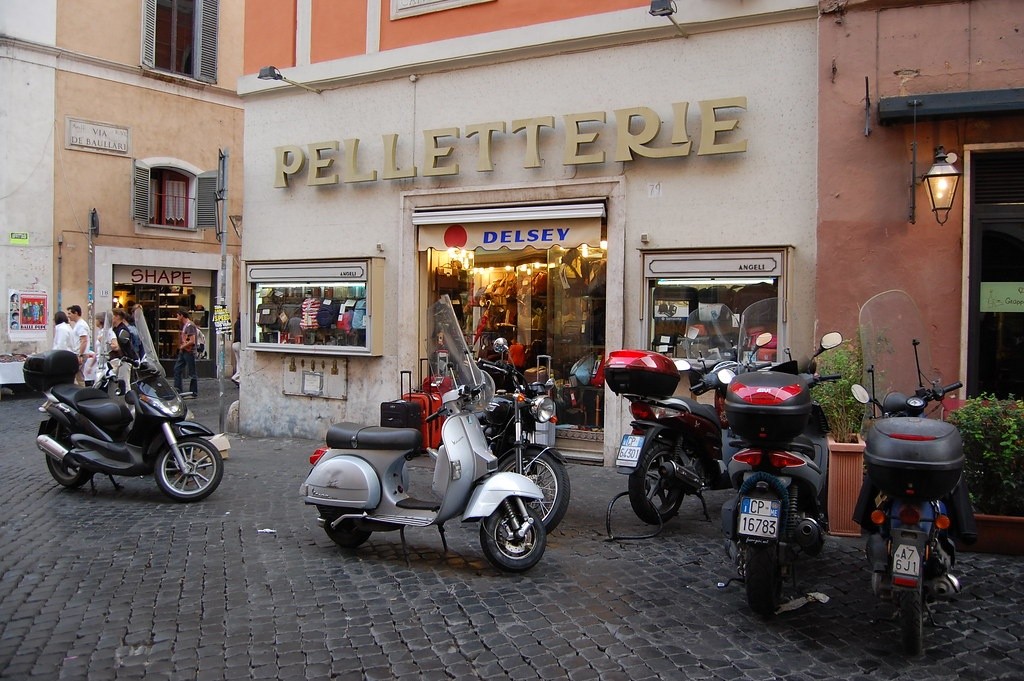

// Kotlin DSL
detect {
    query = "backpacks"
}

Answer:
[560,377,585,425]
[183,322,205,353]
[298,297,366,337]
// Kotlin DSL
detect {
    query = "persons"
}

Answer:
[173,309,198,399]
[231,311,240,387]
[39,303,147,412]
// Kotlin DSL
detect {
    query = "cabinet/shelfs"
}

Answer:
[155,293,182,345]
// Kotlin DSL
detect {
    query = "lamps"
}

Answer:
[648,0,689,38]
[921,145,963,226]
[257,65,321,95]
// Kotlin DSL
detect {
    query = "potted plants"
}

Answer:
[808,318,899,538]
[946,392,1024,554]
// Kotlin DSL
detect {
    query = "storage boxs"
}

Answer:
[862,417,966,504]
[603,350,679,398]
[724,371,814,445]
[22,350,80,391]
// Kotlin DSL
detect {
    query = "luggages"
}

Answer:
[381,349,557,448]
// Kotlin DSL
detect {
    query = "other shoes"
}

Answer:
[183,394,197,399]
[231,378,239,386]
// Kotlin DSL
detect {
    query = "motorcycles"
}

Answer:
[297,293,547,570]
[602,308,771,528]
[850,288,970,658]
[23,305,223,503]
[465,336,571,534]
[690,294,842,617]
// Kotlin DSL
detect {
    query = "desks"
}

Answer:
[0,353,37,384]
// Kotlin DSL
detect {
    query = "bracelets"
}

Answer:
[79,355,83,357]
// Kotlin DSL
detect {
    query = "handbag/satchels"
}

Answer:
[268,303,290,332]
[436,258,548,301]
[257,296,278,325]
